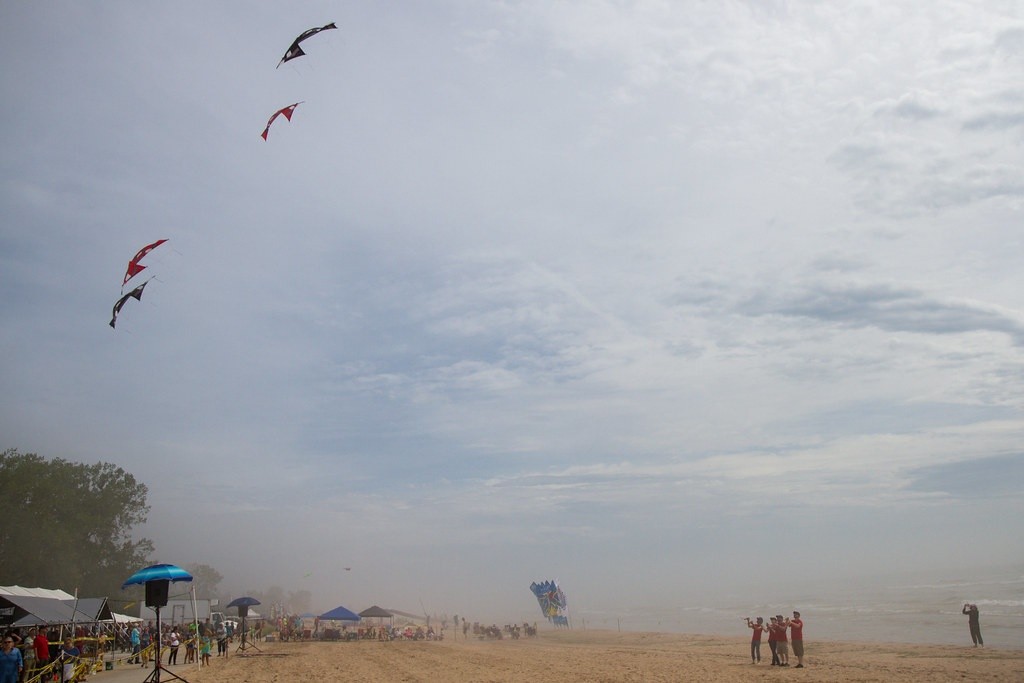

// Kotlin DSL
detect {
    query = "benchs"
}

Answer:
[266,634,276,642]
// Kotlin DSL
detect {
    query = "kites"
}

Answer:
[528,582,569,630]
[125,238,169,286]
[276,21,340,69]
[110,271,159,329]
[261,102,304,140]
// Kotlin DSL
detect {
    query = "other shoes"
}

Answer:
[127,660,133,664]
[136,661,141,663]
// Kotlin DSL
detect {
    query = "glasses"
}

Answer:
[4,641,14,644]
[65,642,70,645]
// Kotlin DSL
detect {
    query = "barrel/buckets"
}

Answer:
[105,662,112,670]
[63,664,74,683]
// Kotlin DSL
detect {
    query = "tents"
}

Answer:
[100,614,143,631]
[359,604,395,629]
[320,607,362,631]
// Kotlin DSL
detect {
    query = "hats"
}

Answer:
[133,623,139,627]
[28,627,36,632]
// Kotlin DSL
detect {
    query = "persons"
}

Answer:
[963,604,984,650]
[743,611,804,669]
[0,614,539,683]
